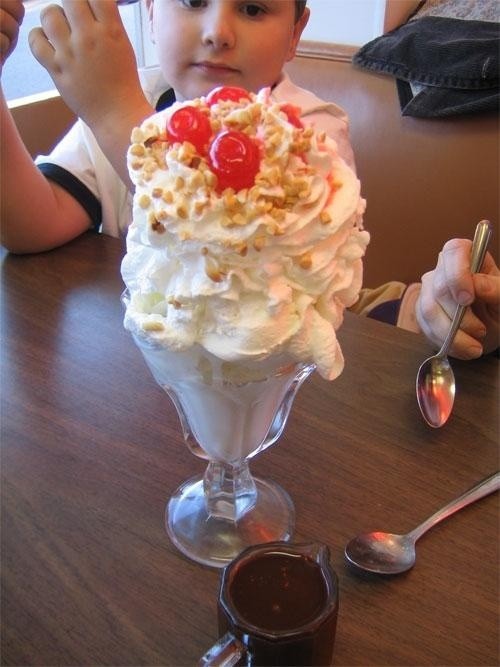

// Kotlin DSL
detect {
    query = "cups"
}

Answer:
[192,540,341,667]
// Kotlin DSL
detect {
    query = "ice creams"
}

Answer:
[121,87,369,465]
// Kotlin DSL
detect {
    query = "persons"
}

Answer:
[1,1,364,312]
[413,236,499,362]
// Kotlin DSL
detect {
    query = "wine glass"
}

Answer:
[120,289,318,566]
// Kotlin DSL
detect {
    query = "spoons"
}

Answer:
[414,217,492,430]
[343,471,500,574]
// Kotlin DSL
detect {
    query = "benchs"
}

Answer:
[284,42,500,323]
